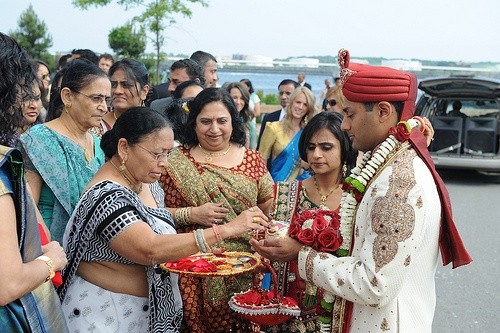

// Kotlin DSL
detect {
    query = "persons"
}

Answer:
[254,72,360,184]
[146,51,262,150]
[265,110,360,333]
[0,31,73,333]
[447,100,468,117]
[9,48,159,245]
[57,106,268,333]
[250,48,472,333]
[159,87,275,333]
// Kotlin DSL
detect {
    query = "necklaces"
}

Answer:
[198,143,231,163]
[113,112,118,120]
[312,176,341,209]
[59,116,91,162]
[109,160,143,194]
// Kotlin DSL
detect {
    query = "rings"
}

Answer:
[248,208,253,212]
[253,217,258,223]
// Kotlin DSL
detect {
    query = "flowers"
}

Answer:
[288,207,343,254]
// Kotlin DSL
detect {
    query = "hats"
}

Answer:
[338,49,417,102]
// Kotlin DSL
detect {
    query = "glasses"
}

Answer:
[70,89,112,104]
[127,142,173,161]
[325,100,338,107]
[38,73,50,80]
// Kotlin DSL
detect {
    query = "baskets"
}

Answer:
[227,262,301,325]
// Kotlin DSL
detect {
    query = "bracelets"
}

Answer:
[35,255,55,282]
[193,230,202,251]
[200,229,212,251]
[174,207,193,226]
[212,223,223,241]
[196,229,207,253]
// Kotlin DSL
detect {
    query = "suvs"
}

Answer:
[413,73,500,179]
